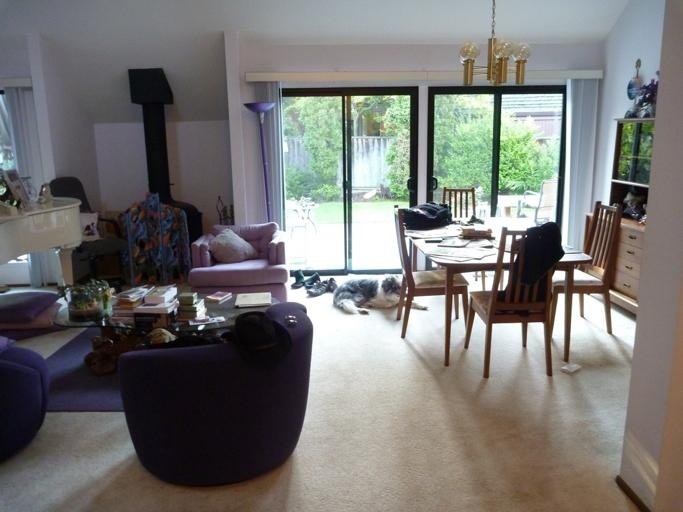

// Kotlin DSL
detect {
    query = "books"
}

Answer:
[402,223,495,261]
[112,284,273,318]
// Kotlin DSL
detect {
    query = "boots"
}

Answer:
[303,271,321,288]
[290,269,305,289]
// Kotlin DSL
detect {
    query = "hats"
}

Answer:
[219,309,292,361]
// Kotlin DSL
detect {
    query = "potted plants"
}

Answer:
[58,276,110,317]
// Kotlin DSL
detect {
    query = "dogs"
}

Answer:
[333,275,431,315]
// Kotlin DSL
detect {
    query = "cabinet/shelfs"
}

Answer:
[582,212,646,314]
[611,116,656,188]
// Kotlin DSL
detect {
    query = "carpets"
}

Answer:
[38,322,127,412]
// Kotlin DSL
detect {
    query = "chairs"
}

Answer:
[516,180,559,221]
[1,332,52,469]
[543,200,625,341]
[186,221,290,301]
[392,203,472,338]
[442,186,478,281]
[48,175,127,286]
[461,225,560,379]
[117,296,312,488]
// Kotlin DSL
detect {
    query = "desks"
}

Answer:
[0,195,83,287]
[405,215,596,368]
[498,195,537,217]
[286,201,319,235]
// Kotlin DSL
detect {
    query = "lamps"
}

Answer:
[243,101,281,223]
[456,1,531,88]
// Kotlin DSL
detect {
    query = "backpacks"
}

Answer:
[401,201,453,232]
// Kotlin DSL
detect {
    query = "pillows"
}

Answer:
[0,300,63,332]
[0,322,64,343]
[78,211,103,242]
[94,234,129,257]
[0,289,58,325]
[210,228,258,265]
